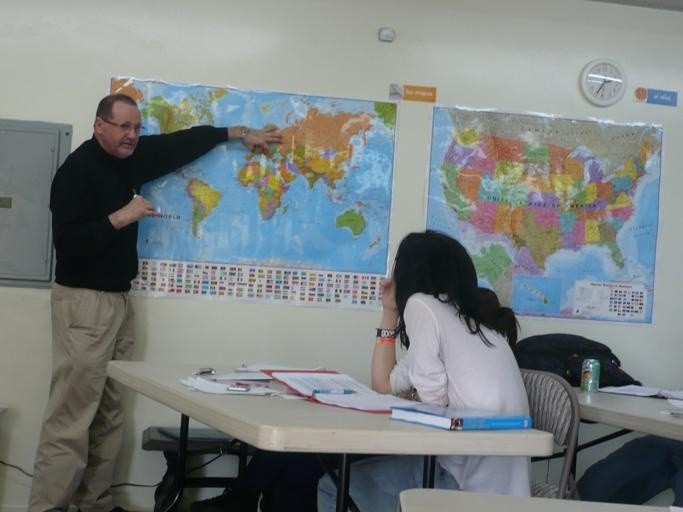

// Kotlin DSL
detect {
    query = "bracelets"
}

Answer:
[240,127,249,139]
[405,388,416,401]
[375,328,397,345]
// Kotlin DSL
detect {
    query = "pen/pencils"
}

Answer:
[312,389,357,394]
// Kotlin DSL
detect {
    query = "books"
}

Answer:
[261,369,422,412]
[389,404,529,431]
[269,381,301,395]
[597,384,683,417]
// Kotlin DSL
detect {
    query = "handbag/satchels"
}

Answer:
[513,334,634,386]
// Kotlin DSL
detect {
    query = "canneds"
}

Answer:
[580,358,601,392]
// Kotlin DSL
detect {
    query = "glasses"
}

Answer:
[103,118,145,133]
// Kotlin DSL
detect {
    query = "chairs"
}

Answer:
[522,367,580,496]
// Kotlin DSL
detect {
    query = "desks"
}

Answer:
[395,485,681,512]
[568,387,682,505]
[108,356,555,512]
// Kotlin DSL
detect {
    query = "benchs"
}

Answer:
[138,423,253,509]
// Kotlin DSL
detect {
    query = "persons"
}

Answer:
[27,93,282,512]
[371,229,532,495]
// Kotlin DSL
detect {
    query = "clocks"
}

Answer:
[580,59,626,108]
